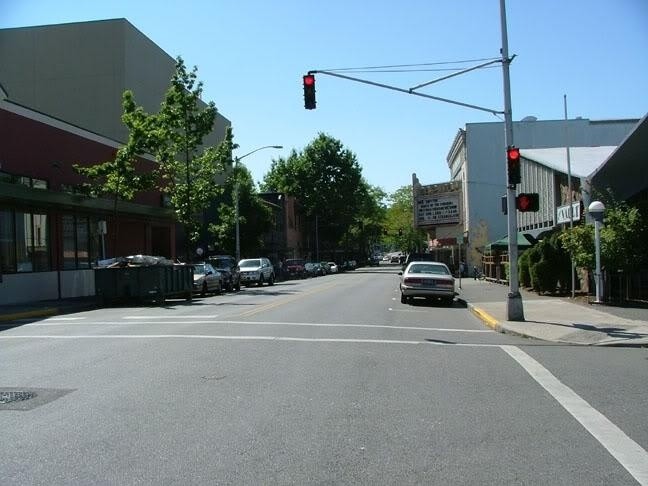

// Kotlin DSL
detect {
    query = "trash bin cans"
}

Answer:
[93,264,196,306]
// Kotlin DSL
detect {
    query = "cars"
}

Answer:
[368,251,456,306]
[189,255,276,298]
[286,258,356,279]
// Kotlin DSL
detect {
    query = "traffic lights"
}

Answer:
[399,228,402,235]
[384,228,387,234]
[506,145,521,186]
[515,191,540,213]
[303,73,317,109]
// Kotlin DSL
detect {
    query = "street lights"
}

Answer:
[588,201,606,301]
[234,145,283,263]
